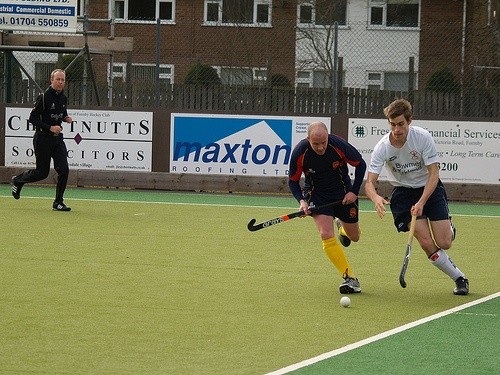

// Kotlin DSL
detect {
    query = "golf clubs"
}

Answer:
[399,206,417,289]
[248,199,355,233]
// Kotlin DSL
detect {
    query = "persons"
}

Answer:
[11,69,73,212]
[365,97,470,296]
[288,122,367,293]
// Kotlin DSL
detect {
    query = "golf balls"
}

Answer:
[340,296,352,306]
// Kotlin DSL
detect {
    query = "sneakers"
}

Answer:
[448,216,456,240]
[453,277,470,294]
[52,200,72,211]
[335,217,351,247]
[339,277,361,293]
[11,175,22,199]
